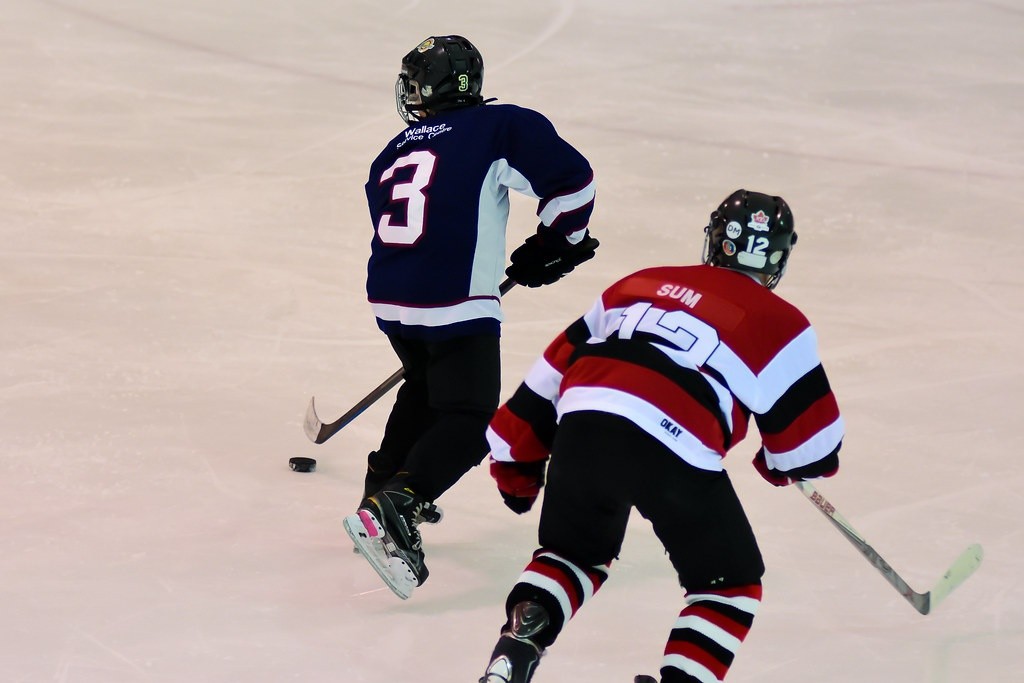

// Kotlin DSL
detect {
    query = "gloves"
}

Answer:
[497,457,547,514]
[504,222,600,288]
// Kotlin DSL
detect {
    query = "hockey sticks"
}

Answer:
[302,236,601,447]
[790,477,990,621]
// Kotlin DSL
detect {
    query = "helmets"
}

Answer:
[702,190,797,291]
[394,35,484,126]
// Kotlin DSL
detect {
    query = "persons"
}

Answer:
[342,35,597,600]
[478,189,844,683]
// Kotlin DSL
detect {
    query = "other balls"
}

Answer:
[287,456,318,474]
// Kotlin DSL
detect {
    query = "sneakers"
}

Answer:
[349,450,387,554]
[480,601,550,683]
[344,475,443,600]
